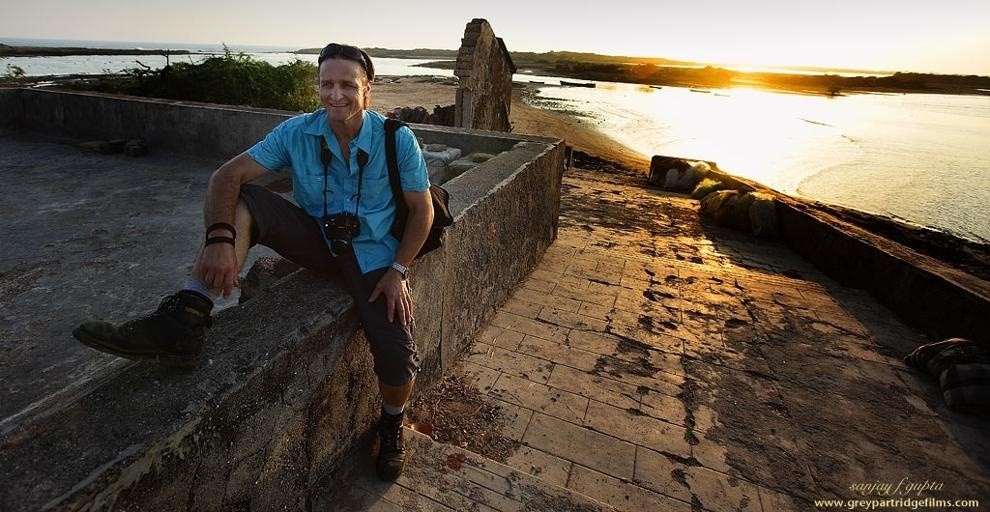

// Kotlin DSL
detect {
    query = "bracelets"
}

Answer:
[205,237,235,248]
[206,223,236,240]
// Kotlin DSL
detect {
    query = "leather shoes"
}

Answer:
[69,290,213,384]
[375,406,407,481]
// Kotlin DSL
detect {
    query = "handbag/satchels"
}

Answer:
[383,120,453,260]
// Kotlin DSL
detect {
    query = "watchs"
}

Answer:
[392,262,410,279]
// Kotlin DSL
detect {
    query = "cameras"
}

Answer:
[323,213,359,255]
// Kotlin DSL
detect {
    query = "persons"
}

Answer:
[71,43,434,481]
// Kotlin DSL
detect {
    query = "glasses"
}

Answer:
[318,43,365,68]
